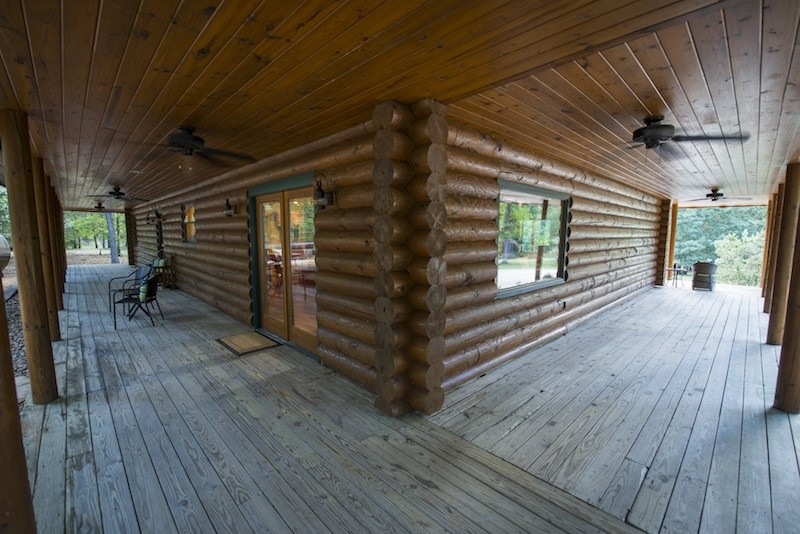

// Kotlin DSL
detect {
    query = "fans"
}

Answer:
[602,115,750,166]
[86,187,149,203]
[684,184,753,206]
[113,126,259,175]
[86,201,116,213]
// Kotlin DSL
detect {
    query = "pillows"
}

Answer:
[151,255,169,272]
[139,276,159,302]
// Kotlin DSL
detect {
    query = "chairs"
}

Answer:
[691,262,718,292]
[109,264,153,315]
[147,253,175,289]
[112,268,165,330]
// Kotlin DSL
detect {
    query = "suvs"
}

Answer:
[292,242,317,256]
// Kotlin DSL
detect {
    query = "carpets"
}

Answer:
[215,331,282,358]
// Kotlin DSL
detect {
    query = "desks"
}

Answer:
[666,268,688,288]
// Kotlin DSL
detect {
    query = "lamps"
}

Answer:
[313,175,333,211]
[146,214,155,225]
[156,210,165,222]
[225,198,237,218]
